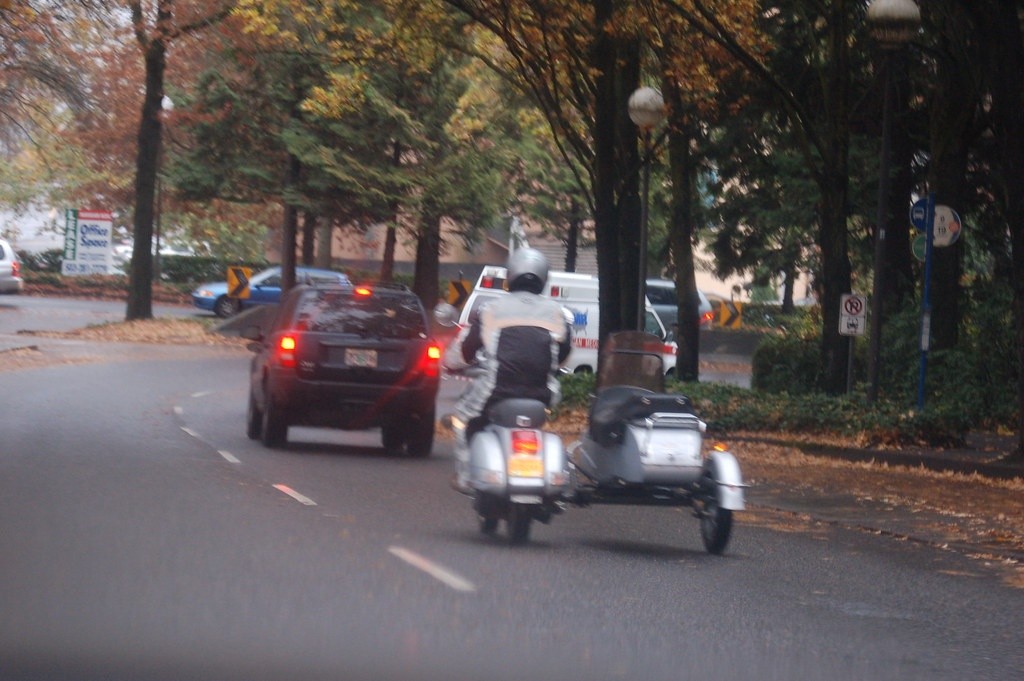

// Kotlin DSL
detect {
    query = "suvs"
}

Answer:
[238,282,447,461]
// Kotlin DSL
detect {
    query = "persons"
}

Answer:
[444,247,572,517]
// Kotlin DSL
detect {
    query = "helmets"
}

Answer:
[507,247,548,291]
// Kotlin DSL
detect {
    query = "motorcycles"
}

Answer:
[432,245,749,558]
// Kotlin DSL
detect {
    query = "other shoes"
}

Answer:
[451,474,476,498]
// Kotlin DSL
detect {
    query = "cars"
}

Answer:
[642,276,715,338]
[192,263,354,320]
[459,270,679,391]
[0,236,26,296]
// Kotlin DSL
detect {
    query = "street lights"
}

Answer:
[629,86,666,338]
[154,95,174,313]
[864,1,948,415]
